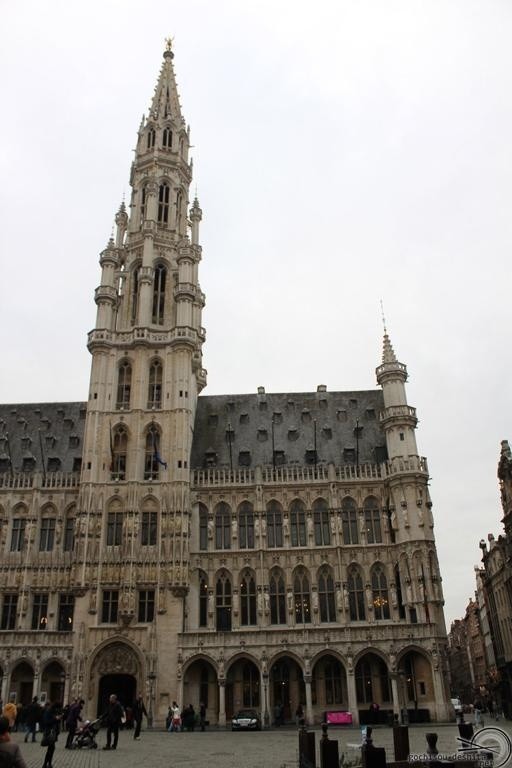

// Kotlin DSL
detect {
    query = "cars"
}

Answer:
[451,699,462,716]
[231,708,260,731]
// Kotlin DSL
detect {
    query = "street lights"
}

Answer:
[146,670,156,730]
[262,670,271,729]
[398,668,409,726]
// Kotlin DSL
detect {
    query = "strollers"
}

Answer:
[69,713,108,749]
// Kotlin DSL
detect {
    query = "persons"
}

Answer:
[272,700,305,729]
[0,692,208,768]
[474,694,500,722]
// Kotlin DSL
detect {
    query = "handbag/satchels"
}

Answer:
[172,718,182,728]
[40,728,56,747]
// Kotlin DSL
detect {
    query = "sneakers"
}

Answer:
[65,744,116,751]
[133,736,141,741]
[23,739,36,742]
